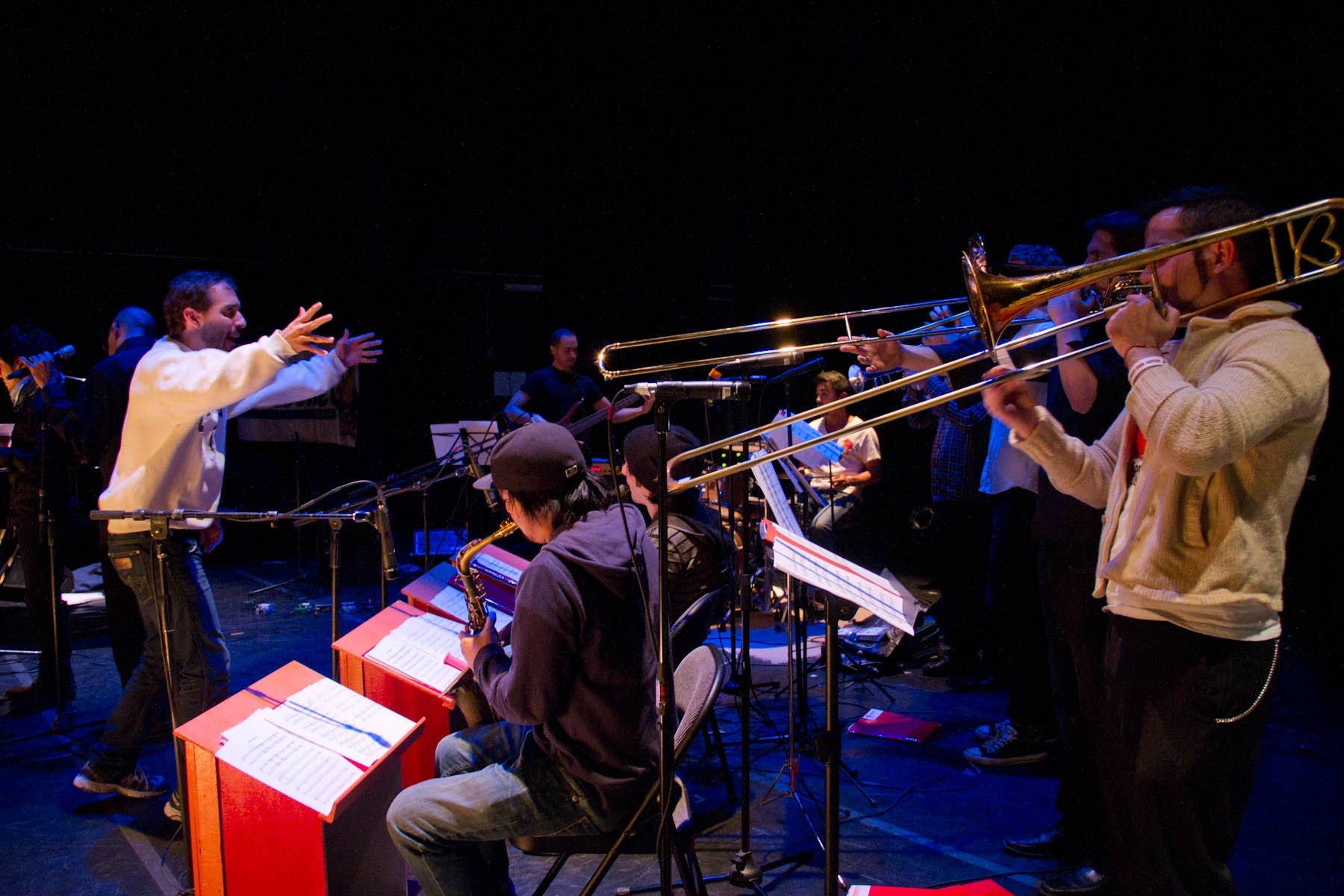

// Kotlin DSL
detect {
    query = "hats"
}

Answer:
[489,422,588,490]
[623,424,704,501]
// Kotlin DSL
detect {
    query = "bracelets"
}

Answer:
[1123,344,1169,384]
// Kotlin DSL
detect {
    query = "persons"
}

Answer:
[386,423,659,896]
[0,307,174,743]
[505,328,655,471]
[622,423,734,666]
[838,209,1147,896]
[982,186,1330,896]
[806,370,881,550]
[73,270,382,822]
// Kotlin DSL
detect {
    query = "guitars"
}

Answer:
[554,374,671,448]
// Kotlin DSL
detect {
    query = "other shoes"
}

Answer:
[142,710,175,743]
[4,676,77,702]
[922,650,982,675]
[948,663,1016,691]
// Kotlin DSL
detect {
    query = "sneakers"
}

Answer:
[164,786,183,822]
[963,722,1048,764]
[975,716,1061,742]
[74,759,171,798]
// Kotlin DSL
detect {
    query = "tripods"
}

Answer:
[613,391,895,896]
[0,398,111,755]
[246,432,334,597]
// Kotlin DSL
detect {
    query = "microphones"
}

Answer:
[722,376,768,383]
[722,350,805,368]
[624,381,753,403]
[468,448,500,512]
[377,491,400,580]
[6,345,76,380]
[770,358,826,384]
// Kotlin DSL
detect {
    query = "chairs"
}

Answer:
[506,640,725,896]
[664,582,738,832]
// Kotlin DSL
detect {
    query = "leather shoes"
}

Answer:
[1038,862,1117,895]
[1004,820,1102,858]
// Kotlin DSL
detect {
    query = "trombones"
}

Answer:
[662,192,1344,496]
[592,231,1109,384]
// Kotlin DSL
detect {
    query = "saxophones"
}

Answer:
[437,517,522,729]
